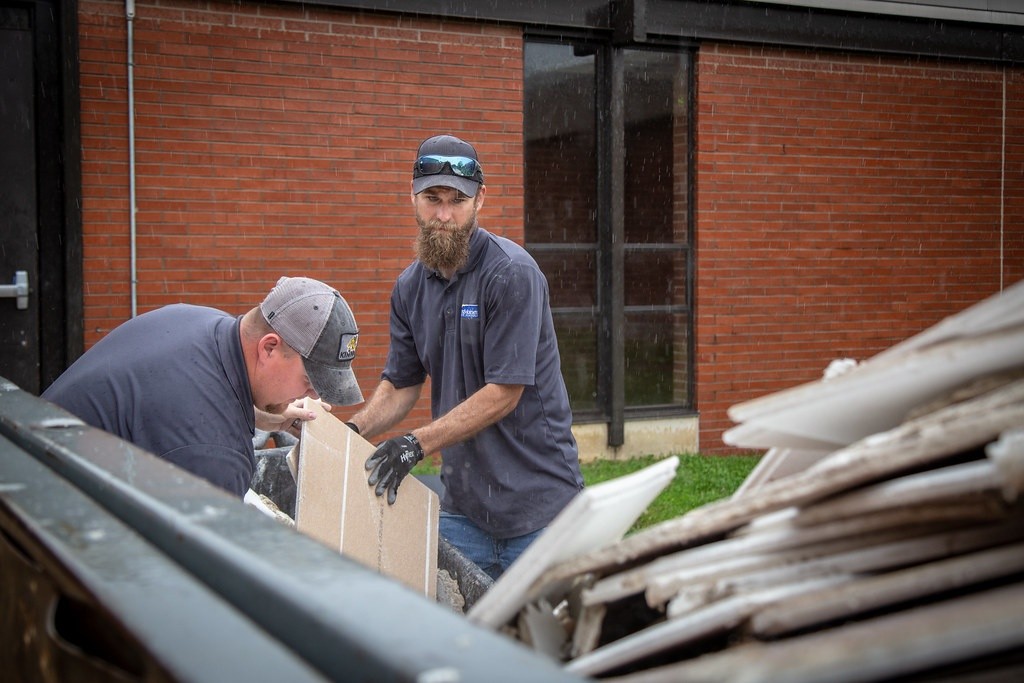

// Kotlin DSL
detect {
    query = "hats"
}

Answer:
[259,276,365,406]
[411,133,483,197]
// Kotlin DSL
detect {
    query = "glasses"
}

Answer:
[412,153,483,178]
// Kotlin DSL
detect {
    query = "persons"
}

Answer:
[40,276,365,503]
[342,133,584,581]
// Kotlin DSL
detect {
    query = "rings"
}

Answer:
[293,419,300,427]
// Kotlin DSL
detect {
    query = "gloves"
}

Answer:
[364,433,425,504]
